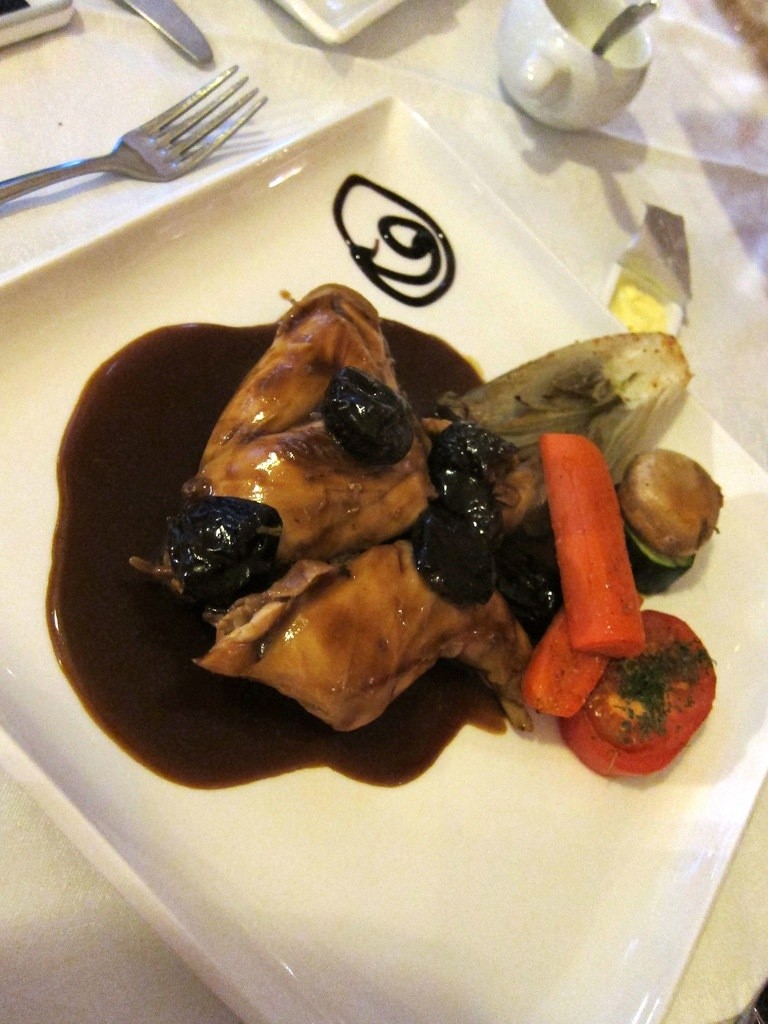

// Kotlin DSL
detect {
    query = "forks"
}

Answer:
[0,64,270,207]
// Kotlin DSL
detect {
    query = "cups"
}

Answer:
[496,0,653,132]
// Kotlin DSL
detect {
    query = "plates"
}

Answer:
[272,0,406,47]
[0,94,767,1024]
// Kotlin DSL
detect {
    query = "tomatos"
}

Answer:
[552,611,716,778]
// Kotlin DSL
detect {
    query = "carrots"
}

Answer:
[539,432,645,659]
[521,589,647,718]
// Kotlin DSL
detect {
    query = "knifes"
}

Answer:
[123,0,214,63]
